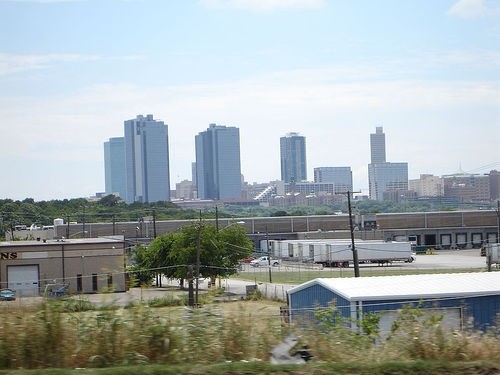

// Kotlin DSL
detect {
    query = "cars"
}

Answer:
[0,288,16,301]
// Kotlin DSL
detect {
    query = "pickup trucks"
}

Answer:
[249,255,282,267]
